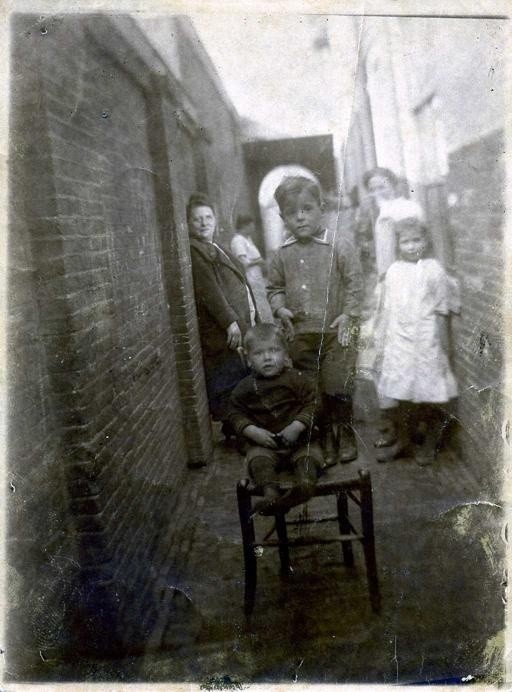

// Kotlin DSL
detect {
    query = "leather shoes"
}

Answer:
[323,428,358,466]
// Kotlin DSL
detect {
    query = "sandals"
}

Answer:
[374,433,437,465]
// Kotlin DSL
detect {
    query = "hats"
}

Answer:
[189,192,212,205]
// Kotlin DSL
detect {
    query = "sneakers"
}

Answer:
[255,483,311,517]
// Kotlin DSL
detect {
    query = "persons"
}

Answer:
[185,190,262,446]
[229,209,275,324]
[224,321,324,516]
[281,165,430,423]
[265,174,367,465]
[373,217,462,466]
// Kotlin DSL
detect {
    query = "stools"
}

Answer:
[235,463,380,616]
